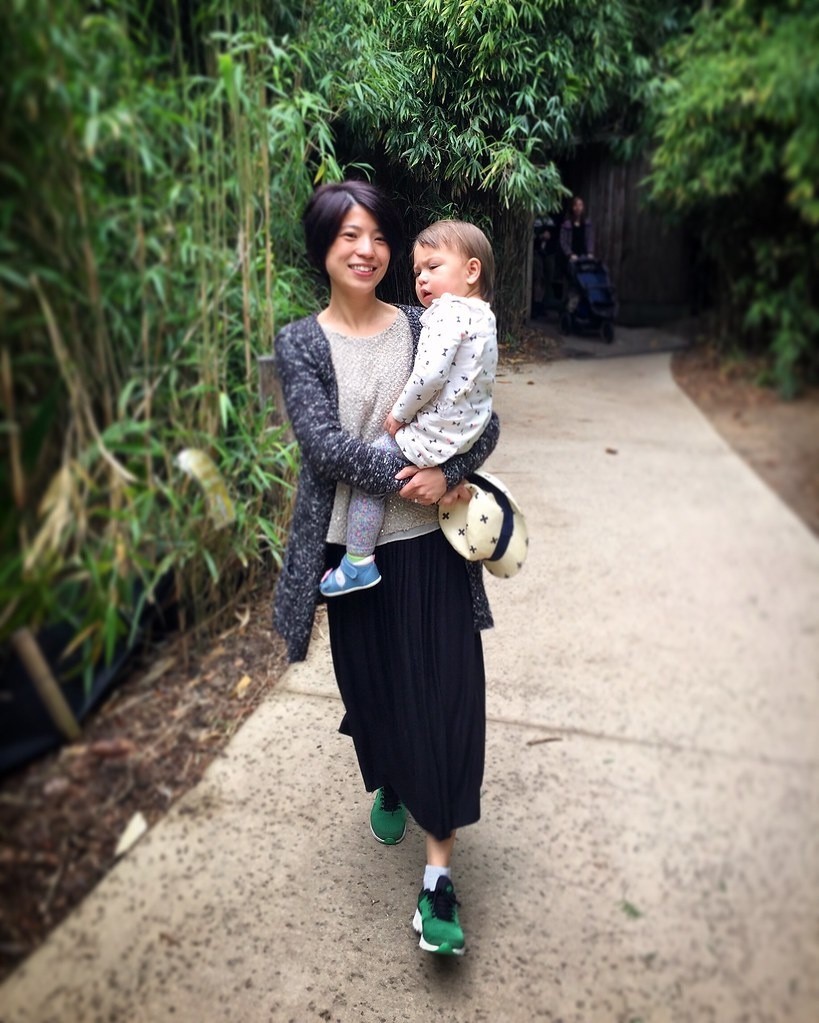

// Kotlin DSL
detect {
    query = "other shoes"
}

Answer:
[318,554,381,596]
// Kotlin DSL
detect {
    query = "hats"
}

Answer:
[438,471,529,577]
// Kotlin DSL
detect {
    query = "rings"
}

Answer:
[415,498,418,503]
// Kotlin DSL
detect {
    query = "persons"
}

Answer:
[269,182,500,957]
[320,218,499,597]
[559,197,595,312]
[535,214,556,297]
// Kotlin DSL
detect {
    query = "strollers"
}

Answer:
[560,257,618,344]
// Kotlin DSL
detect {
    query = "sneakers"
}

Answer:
[412,875,466,955]
[370,787,407,843]
